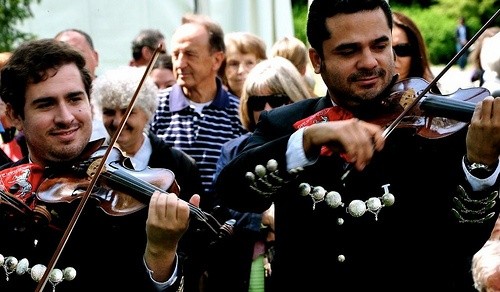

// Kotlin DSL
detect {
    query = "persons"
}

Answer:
[471,238,500,291]
[55,29,111,146]
[147,14,247,193]
[91,66,205,292]
[0,52,27,166]
[208,0,500,292]
[389,9,441,89]
[128,29,166,67]
[454,14,470,70]
[468,26,500,96]
[151,54,177,89]
[272,36,318,99]
[219,32,268,99]
[0,38,201,292]
[209,56,314,292]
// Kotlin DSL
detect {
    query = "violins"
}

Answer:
[358,77,491,156]
[36,146,236,241]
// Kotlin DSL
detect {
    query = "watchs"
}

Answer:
[464,155,499,179]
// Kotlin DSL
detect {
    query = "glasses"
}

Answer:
[392,44,415,57]
[247,93,290,111]
